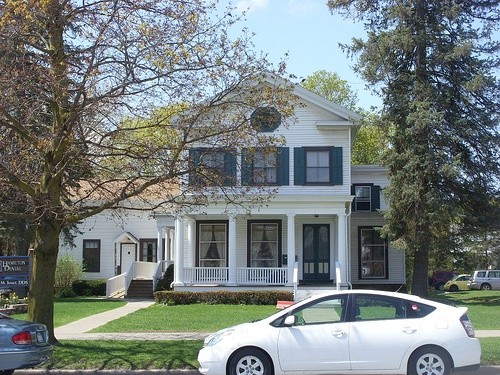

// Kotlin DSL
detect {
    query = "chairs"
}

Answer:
[395,304,405,318]
[355,304,363,321]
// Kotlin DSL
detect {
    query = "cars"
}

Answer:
[444,273,473,293]
[196,289,481,375]
[0,313,54,375]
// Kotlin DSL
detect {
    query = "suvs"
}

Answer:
[429,271,461,290]
[468,269,500,290]
